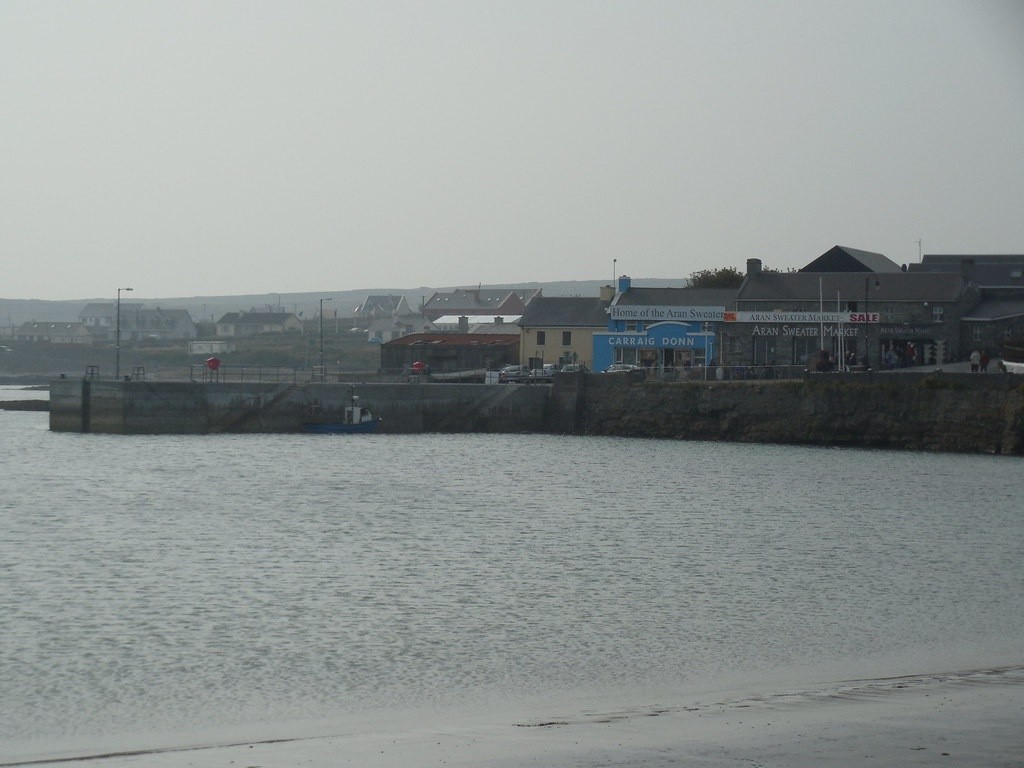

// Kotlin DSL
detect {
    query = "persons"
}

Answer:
[981,350,988,371]
[708,358,716,366]
[886,346,898,368]
[970,350,980,372]
[684,358,691,366]
[906,342,918,367]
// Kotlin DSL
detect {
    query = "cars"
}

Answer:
[531,363,562,384]
[560,362,591,375]
[499,364,531,383]
[600,363,641,373]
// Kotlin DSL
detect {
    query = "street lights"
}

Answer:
[319,297,334,381]
[114,288,135,379]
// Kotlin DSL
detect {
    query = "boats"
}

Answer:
[301,384,383,435]
